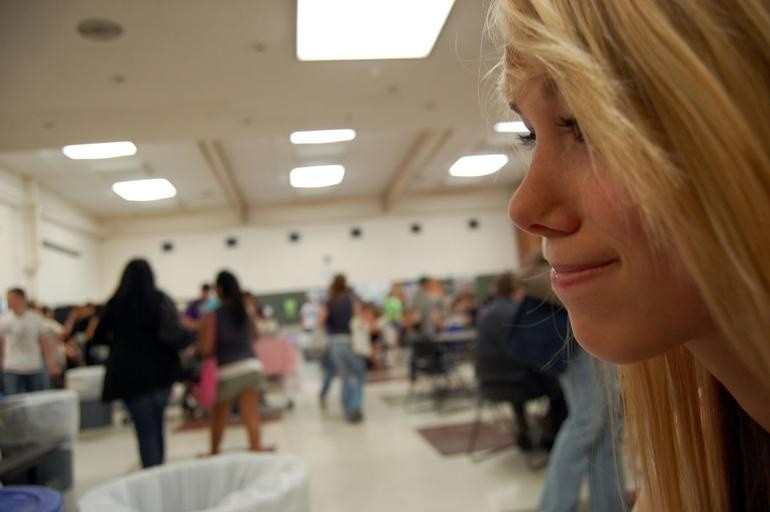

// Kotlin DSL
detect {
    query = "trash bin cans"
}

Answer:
[0,390,80,493]
[75,450,317,512]
[66,365,114,430]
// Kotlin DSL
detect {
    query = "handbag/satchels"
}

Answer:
[349,316,373,356]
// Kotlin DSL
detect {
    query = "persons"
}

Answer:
[362,276,477,374]
[316,274,367,424]
[483,2,770,512]
[175,271,265,456]
[0,287,99,396]
[478,257,576,456]
[92,260,187,473]
